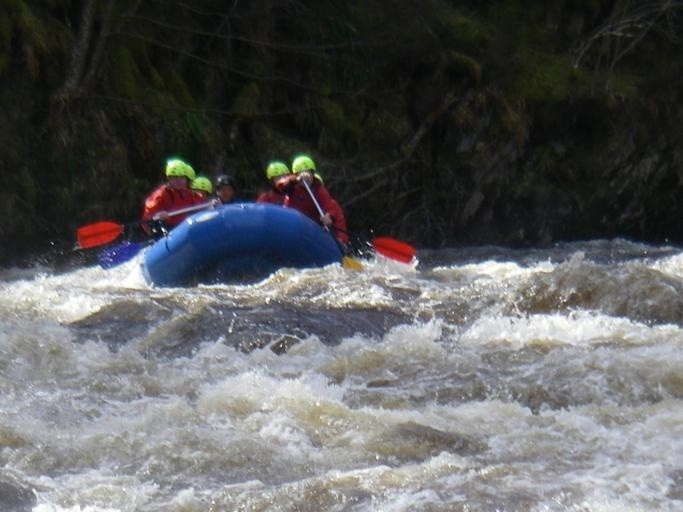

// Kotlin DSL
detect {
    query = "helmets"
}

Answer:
[166,159,196,179]
[216,175,236,187]
[292,156,316,174]
[190,176,213,193]
[267,161,290,179]
[314,173,323,184]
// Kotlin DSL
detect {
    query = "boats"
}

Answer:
[140,203,343,287]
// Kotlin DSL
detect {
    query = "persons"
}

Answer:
[257,155,348,243]
[141,159,236,236]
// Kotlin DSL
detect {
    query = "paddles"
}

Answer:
[303,179,363,270]
[328,223,416,263]
[76,204,213,248]
